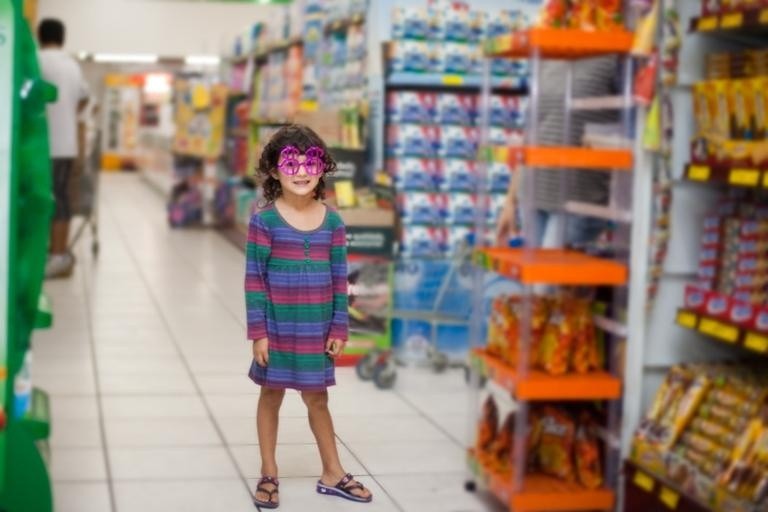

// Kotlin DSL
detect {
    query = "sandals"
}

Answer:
[253,475,280,510]
[316,474,373,502]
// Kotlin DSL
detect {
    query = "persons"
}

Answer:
[243,123,377,509]
[33,16,91,281]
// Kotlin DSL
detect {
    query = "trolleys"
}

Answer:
[351,242,488,393]
[69,127,104,258]
